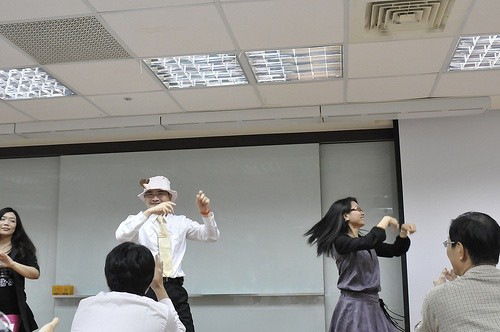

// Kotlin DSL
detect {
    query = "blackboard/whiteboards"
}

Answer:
[52,143,325,298]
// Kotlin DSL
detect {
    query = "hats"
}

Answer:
[138,176,178,203]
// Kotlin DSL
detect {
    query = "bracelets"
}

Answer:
[401,231,408,234]
[200,208,210,214]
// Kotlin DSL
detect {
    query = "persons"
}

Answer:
[0,207,40,332]
[115,175,220,332]
[414,211,500,332]
[302,197,416,332]
[71,242,186,332]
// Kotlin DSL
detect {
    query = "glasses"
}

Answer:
[146,193,167,198]
[443,240,457,248]
[350,206,363,212]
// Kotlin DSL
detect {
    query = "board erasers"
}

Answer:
[52,286,74,295]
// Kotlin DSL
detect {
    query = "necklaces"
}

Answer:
[2,247,12,254]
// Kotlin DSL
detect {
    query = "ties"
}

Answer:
[156,215,173,277]
